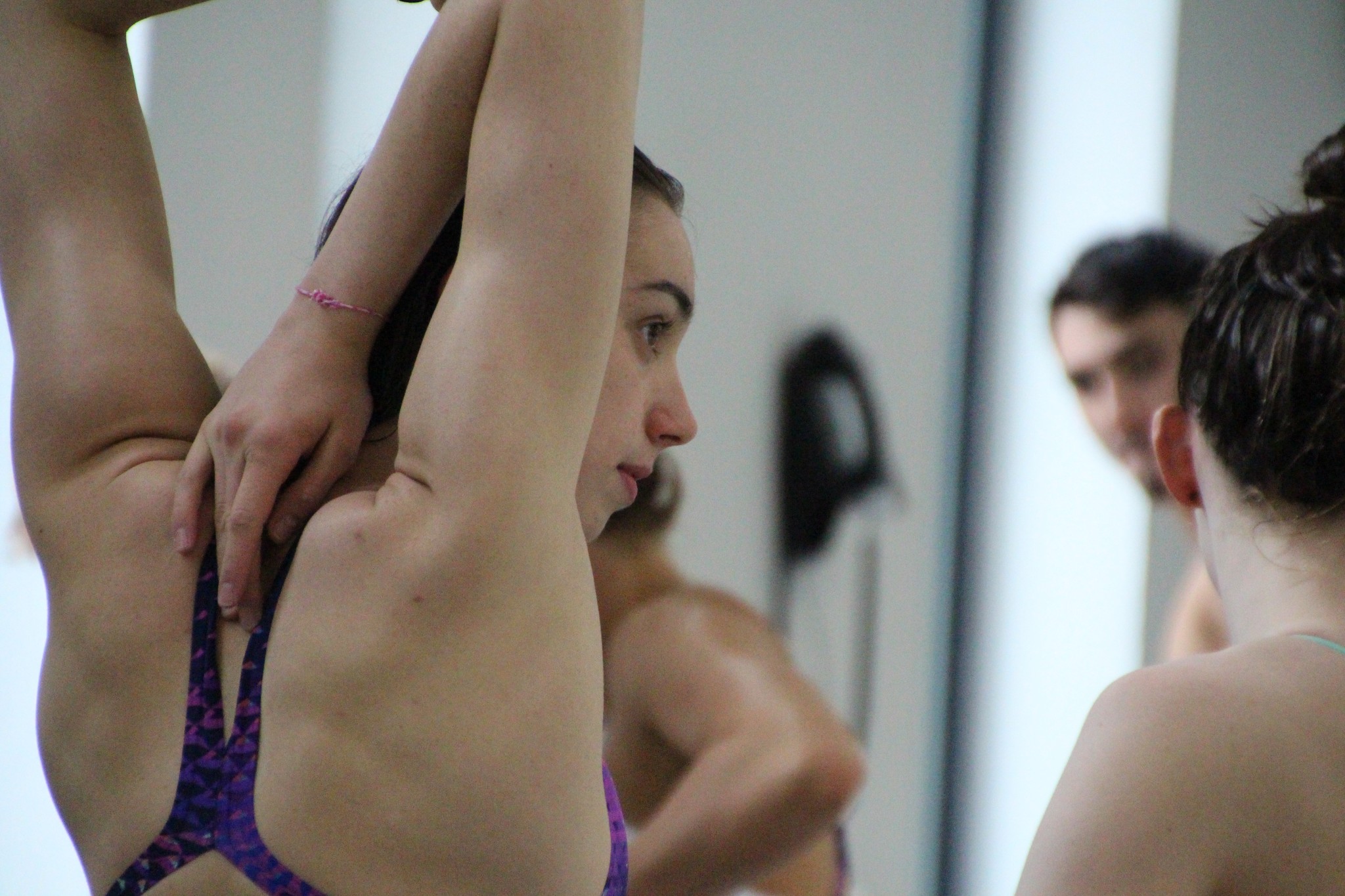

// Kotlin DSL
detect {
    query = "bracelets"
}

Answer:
[293,284,392,323]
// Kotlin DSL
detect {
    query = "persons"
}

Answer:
[588,456,866,896]
[1047,230,1229,662]
[1017,126,1345,896]
[0,0,701,896]
